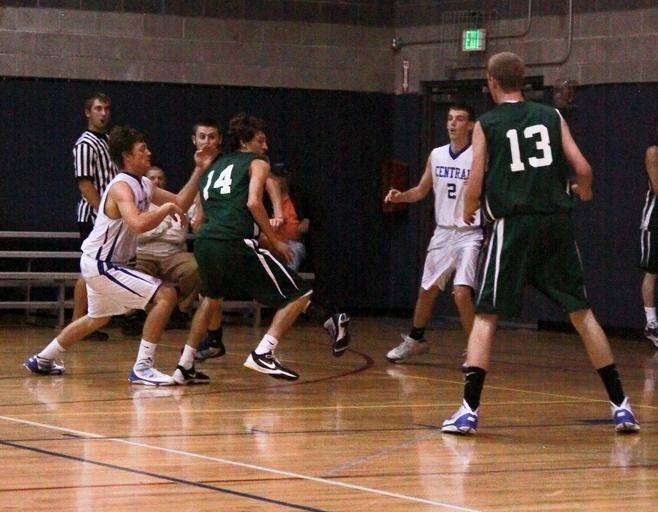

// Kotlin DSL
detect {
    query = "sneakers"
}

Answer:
[127,357,175,386]
[22,355,65,375]
[243,350,299,381]
[181,337,225,359]
[385,333,429,364]
[172,365,210,385]
[644,321,658,348]
[80,330,109,342]
[440,397,480,436]
[323,313,350,357]
[121,317,147,336]
[608,395,640,432]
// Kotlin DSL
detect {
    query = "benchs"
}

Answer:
[0,230,316,328]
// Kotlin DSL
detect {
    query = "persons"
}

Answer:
[442,51,640,436]
[640,145,658,349]
[258,161,316,276]
[23,126,220,386]
[134,166,202,328]
[181,119,350,363]
[72,93,144,341]
[384,105,489,372]
